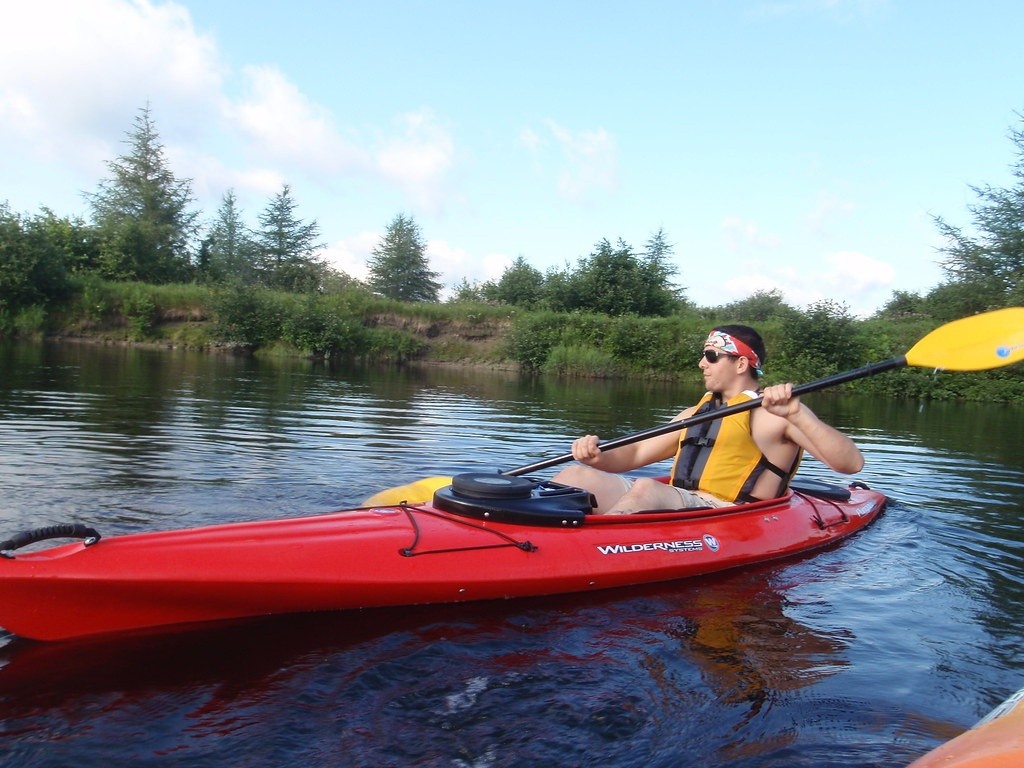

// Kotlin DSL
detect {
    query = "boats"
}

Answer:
[1,470,890,646]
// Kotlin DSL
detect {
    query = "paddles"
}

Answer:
[361,306,1024,509]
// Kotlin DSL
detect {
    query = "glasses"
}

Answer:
[702,349,739,362]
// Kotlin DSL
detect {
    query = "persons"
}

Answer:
[549,324,864,515]
